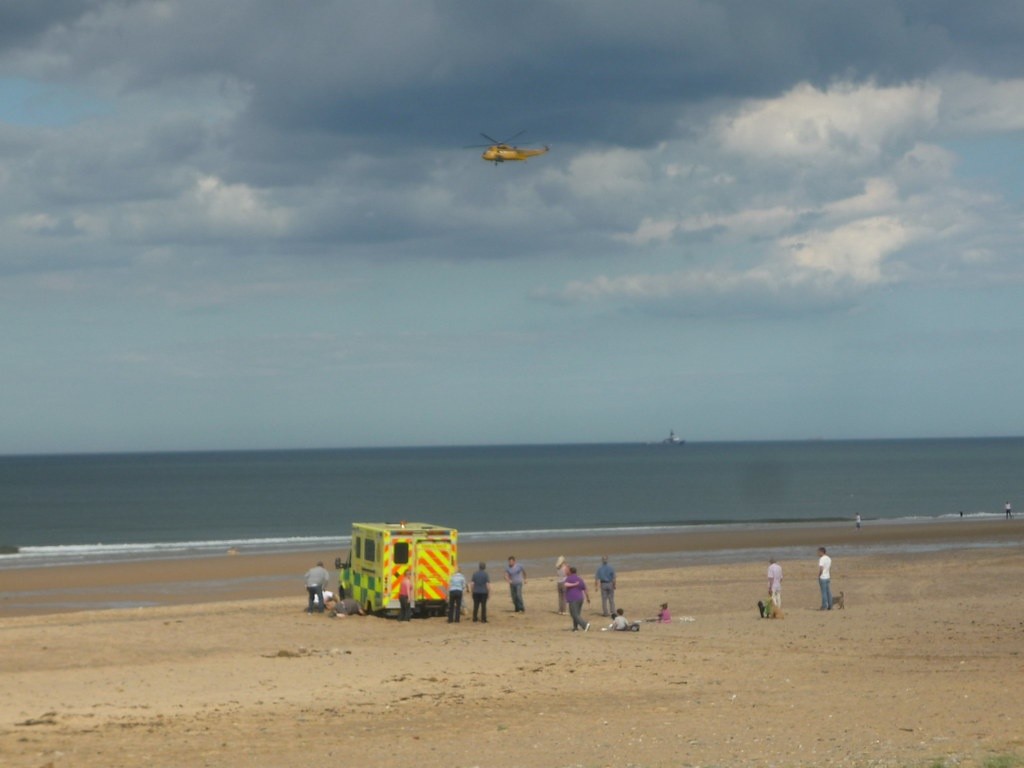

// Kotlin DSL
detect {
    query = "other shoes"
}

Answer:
[572,629,579,632]
[584,622,590,631]
[558,611,566,615]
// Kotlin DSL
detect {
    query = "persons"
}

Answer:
[548,555,570,615]
[817,547,833,610]
[313,589,340,611]
[446,567,469,623]
[503,555,526,614]
[768,558,783,608]
[398,569,413,622]
[855,512,861,529]
[1004,501,1012,520]
[594,554,617,616]
[601,603,671,631]
[331,598,367,618]
[470,561,490,624]
[565,566,592,632]
[302,561,329,614]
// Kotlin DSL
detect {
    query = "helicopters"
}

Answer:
[466,131,552,165]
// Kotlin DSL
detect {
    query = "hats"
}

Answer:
[555,555,565,568]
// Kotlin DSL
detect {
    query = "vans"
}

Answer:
[335,520,457,618]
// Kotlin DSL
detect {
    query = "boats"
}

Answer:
[666,429,685,445]
